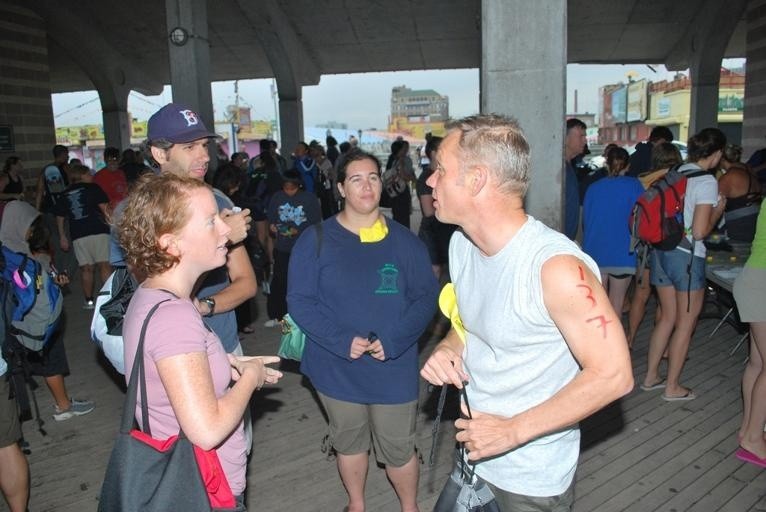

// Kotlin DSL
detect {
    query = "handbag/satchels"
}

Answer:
[428,362,502,512]
[95,300,248,512]
[277,312,308,362]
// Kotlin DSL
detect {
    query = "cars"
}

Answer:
[587,139,688,171]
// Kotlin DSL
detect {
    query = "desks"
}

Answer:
[705,253,750,358]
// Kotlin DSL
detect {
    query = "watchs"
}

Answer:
[200,296,215,318]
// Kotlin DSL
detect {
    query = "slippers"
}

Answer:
[661,387,697,401]
[640,378,666,391]
[737,447,766,470]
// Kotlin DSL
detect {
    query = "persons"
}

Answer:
[248,139,284,296]
[629,126,673,179]
[310,140,319,150]
[285,149,441,512]
[325,130,339,165]
[118,173,283,512]
[580,147,646,323]
[122,146,153,179]
[586,143,620,189]
[391,142,417,231]
[292,142,318,194]
[109,104,260,460]
[565,118,588,244]
[269,141,286,172]
[350,138,359,150]
[379,142,402,209]
[417,134,458,283]
[214,164,256,342]
[231,150,252,196]
[34,144,77,296]
[264,171,323,330]
[134,151,146,165]
[0,156,27,205]
[58,164,112,310]
[312,145,334,219]
[421,113,635,512]
[640,126,728,403]
[0,200,98,422]
[628,143,697,367]
[0,244,30,512]
[331,142,352,203]
[731,187,766,470]
[94,148,130,211]
[712,166,750,214]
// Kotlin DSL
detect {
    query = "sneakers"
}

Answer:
[82,297,95,310]
[53,397,96,421]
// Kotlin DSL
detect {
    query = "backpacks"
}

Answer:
[628,160,717,253]
[91,267,139,393]
[14,252,66,366]
[382,157,406,198]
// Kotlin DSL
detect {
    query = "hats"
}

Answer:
[147,104,223,144]
[63,165,94,183]
